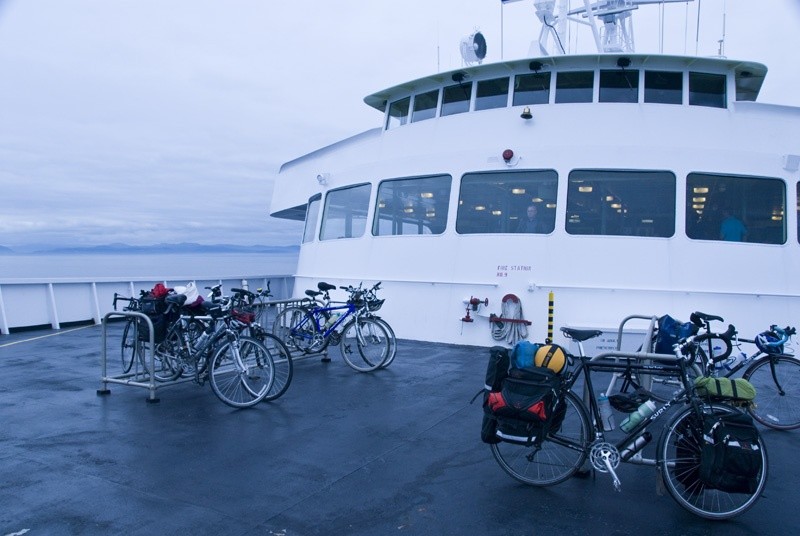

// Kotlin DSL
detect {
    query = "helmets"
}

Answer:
[534,343,568,373]
[367,298,385,312]
[755,329,784,357]
[607,388,651,413]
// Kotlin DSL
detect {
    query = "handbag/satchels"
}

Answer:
[674,413,763,496]
[654,315,698,367]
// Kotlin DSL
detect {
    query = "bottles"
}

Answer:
[325,312,341,328]
[620,399,656,433]
[619,431,652,460]
[192,328,212,353]
[711,346,721,377]
[597,392,615,431]
[337,313,346,333]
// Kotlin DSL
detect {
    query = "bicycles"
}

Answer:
[112,288,293,408]
[271,282,397,372]
[634,311,800,430]
[487,326,769,518]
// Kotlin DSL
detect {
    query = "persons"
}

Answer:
[516,204,548,234]
[720,207,748,242]
